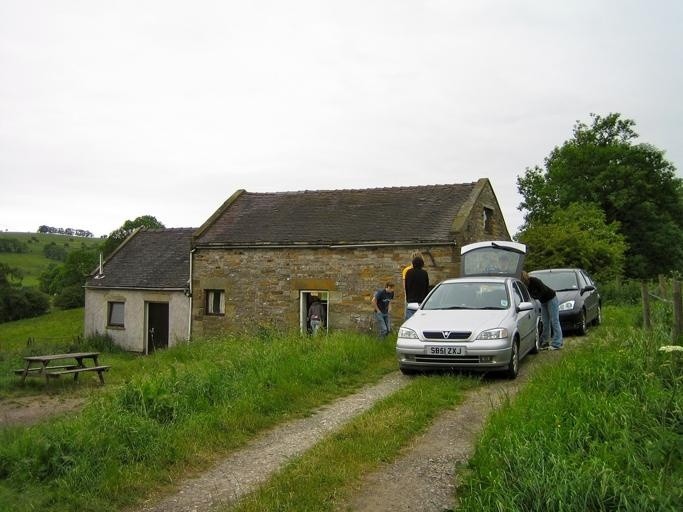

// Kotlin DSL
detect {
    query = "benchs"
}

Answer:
[15,361,79,383]
[46,364,111,390]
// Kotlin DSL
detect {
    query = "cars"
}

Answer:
[393,237,545,381]
[524,266,601,333]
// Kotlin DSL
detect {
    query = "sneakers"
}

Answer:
[539,343,550,349]
[547,345,558,350]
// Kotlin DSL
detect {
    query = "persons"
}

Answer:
[519,271,564,350]
[371,281,396,337]
[405,257,429,320]
[401,250,425,298]
[307,297,325,333]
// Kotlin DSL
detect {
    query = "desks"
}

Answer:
[21,351,106,385]
